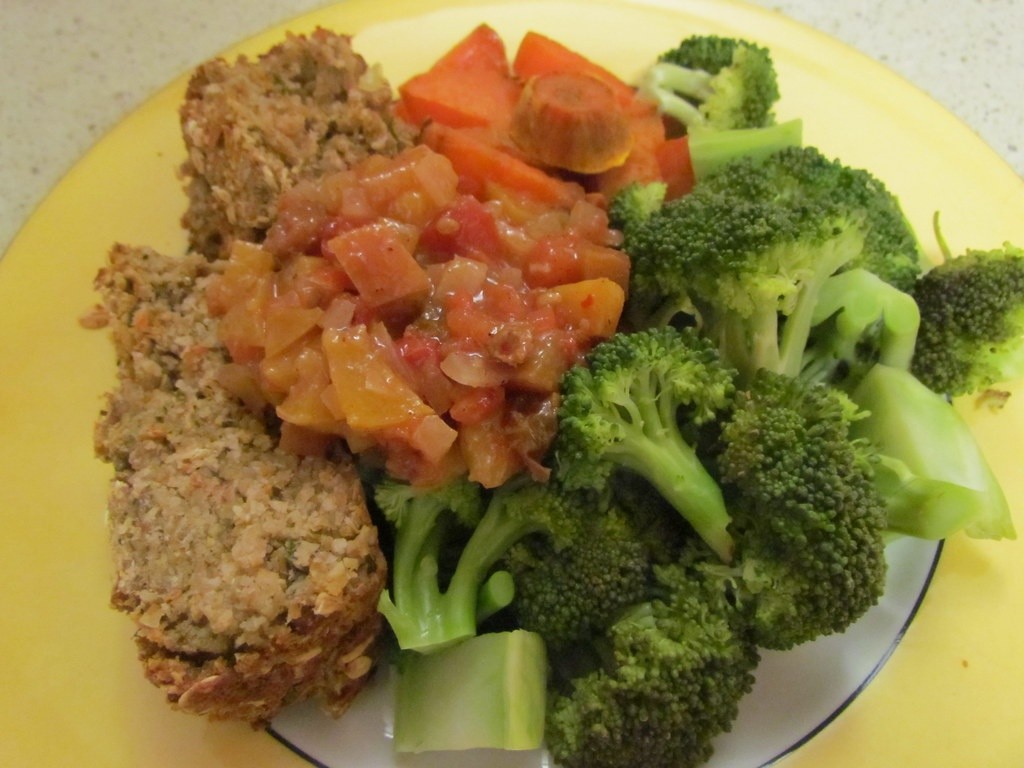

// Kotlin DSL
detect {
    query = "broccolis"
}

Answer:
[373,33,1024,765]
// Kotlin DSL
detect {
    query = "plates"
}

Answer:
[0,0,1024,768]
